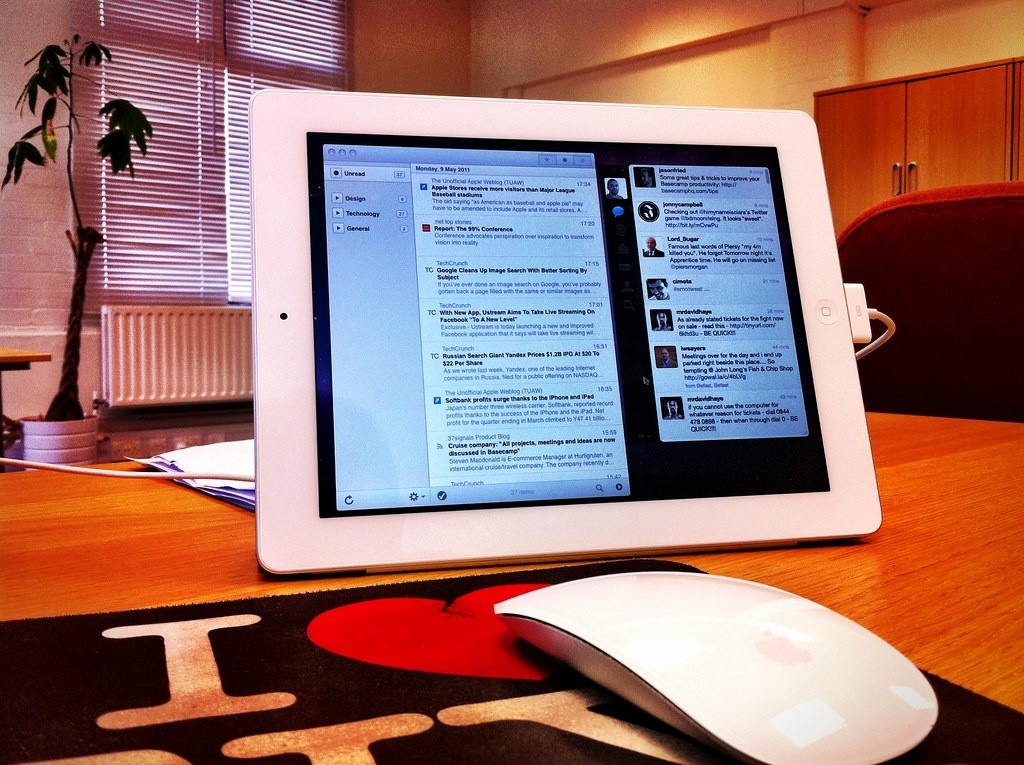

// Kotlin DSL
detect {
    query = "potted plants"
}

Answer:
[0,34,153,472]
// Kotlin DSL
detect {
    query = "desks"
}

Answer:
[0,346,51,473]
[0,411,1024,765]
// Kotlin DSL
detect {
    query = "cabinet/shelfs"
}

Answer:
[1012,56,1024,182]
[814,58,1015,243]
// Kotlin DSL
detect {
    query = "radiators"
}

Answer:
[92,304,251,415]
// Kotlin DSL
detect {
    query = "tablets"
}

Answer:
[248,92,882,577]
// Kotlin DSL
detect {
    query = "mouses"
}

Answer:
[494,572,939,765]
